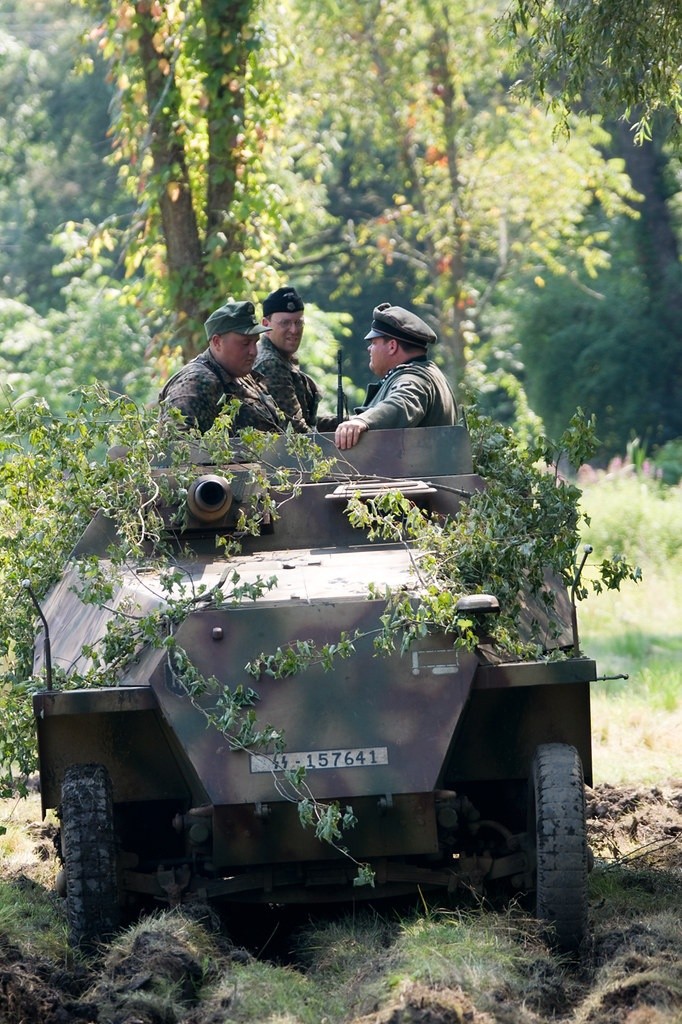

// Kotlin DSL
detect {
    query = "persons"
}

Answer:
[158,301,290,441]
[334,302,457,450]
[252,285,357,433]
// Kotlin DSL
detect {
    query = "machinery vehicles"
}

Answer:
[25,424,628,962]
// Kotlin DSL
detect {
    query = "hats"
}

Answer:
[363,302,437,349]
[205,301,273,342]
[263,287,304,317]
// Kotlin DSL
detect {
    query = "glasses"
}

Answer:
[269,316,305,328]
